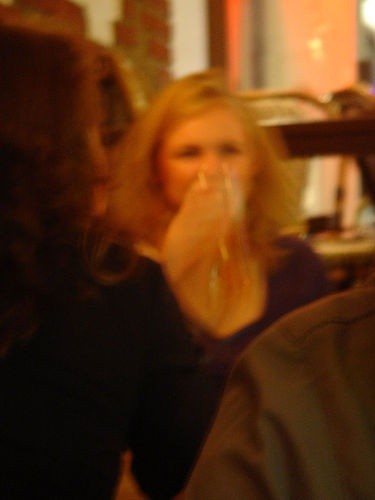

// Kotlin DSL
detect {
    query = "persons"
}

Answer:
[172,91,374,499]
[111,70,330,378]
[0,25,221,499]
